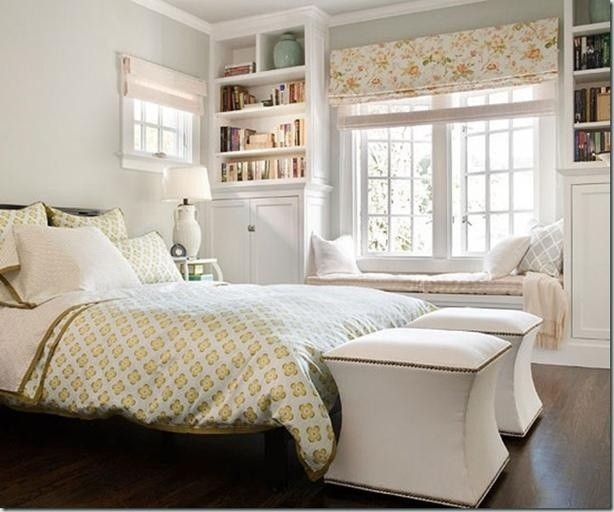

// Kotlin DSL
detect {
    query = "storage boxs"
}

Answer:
[183,274,213,281]
[249,134,275,147]
[597,94,611,120]
[180,264,203,274]
[225,62,254,76]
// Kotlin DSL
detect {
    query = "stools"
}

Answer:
[321,328,512,510]
[403,308,543,437]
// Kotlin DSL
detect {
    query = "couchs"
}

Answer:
[307,274,563,349]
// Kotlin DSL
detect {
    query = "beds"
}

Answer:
[0,204,442,484]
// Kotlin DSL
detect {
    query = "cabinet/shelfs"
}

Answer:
[200,5,334,284]
[555,1,611,348]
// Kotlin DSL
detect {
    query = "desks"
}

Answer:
[173,259,223,282]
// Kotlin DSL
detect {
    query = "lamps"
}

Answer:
[161,166,212,261]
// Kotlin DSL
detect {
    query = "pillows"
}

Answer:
[484,235,532,280]
[0,201,48,309]
[312,232,362,277]
[112,230,184,285]
[511,217,563,278]
[0,222,142,310]
[46,206,128,243]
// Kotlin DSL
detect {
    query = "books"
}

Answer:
[574,34,610,69]
[574,85,610,124]
[574,130,611,160]
[221,122,305,151]
[223,62,254,78]
[222,157,306,183]
[222,81,306,112]
